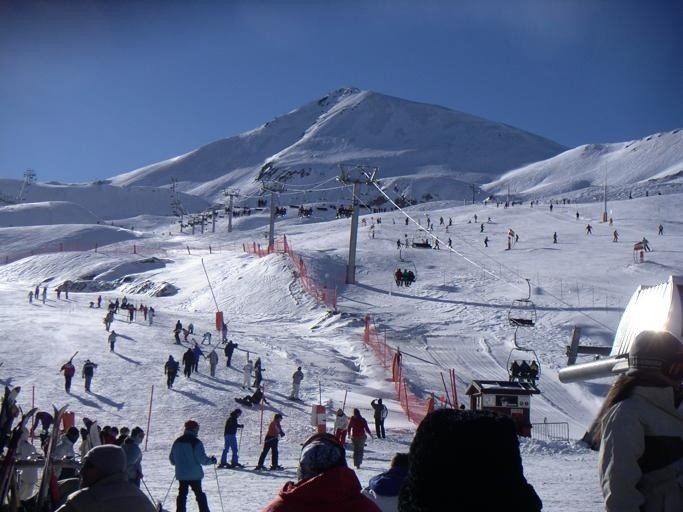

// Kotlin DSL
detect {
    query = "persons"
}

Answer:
[371,398,387,438]
[261,432,382,512]
[361,454,414,512]
[27,282,68,305]
[105,296,155,332]
[218,356,303,471]
[396,233,455,251]
[576,211,580,218]
[82,359,97,392]
[1,377,217,511]
[175,319,228,345]
[506,229,520,250]
[334,408,371,468]
[484,199,540,209]
[90,296,102,307]
[548,198,566,212]
[226,197,267,217]
[107,330,118,352]
[642,238,651,251]
[298,205,314,217]
[554,232,557,243]
[509,359,537,388]
[274,206,287,218]
[373,207,394,213]
[469,214,492,247]
[164,340,238,390]
[658,224,662,235]
[61,361,75,393]
[586,224,592,234]
[376,216,452,230]
[610,218,619,241]
[336,205,355,218]
[597,330,682,512]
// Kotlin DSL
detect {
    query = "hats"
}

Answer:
[297,437,346,479]
[185,420,198,428]
[131,427,143,437]
[84,444,126,473]
[625,331,682,386]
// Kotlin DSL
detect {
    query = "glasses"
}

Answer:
[628,358,682,377]
[300,432,343,457]
